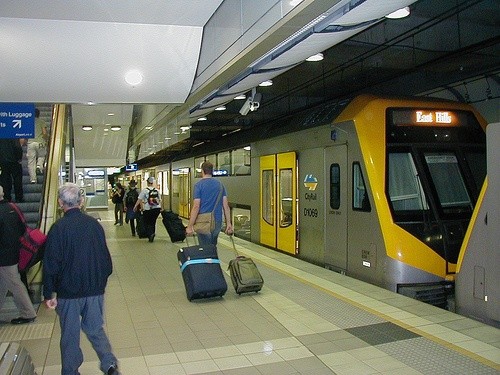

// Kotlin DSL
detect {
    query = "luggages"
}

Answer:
[135,209,148,238]
[224,229,264,296]
[161,209,186,242]
[176,233,228,301]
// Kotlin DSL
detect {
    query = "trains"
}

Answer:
[115,95,500,328]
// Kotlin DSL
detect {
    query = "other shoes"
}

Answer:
[11,316,37,324]
[15,199,26,202]
[36,165,43,174]
[30,180,36,184]
[108,367,118,375]
[0,199,11,203]
[149,234,155,242]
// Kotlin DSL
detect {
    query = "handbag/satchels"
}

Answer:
[193,212,215,234]
[112,194,119,204]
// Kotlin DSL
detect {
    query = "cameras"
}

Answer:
[112,189,118,192]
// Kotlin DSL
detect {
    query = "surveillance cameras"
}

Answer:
[238,92,262,115]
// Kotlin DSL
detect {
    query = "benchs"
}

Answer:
[0,339,38,375]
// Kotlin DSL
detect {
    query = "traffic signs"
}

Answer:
[0,103,35,139]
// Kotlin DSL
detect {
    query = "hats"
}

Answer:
[128,180,137,185]
[146,176,154,184]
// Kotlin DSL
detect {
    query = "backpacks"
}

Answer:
[9,202,47,288]
[128,191,137,205]
[145,188,160,205]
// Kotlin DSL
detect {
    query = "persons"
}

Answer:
[123,180,141,236]
[186,162,233,245]
[42,183,121,374]
[132,177,164,242]
[0,138,24,203]
[24,108,48,183]
[0,185,37,325]
[113,182,125,226]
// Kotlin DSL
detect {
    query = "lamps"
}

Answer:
[251,92,262,108]
[238,97,252,116]
